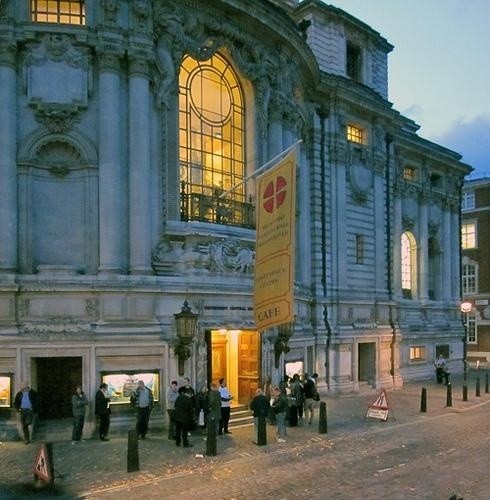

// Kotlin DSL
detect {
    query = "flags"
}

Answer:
[252,147,301,336]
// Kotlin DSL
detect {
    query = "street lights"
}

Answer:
[460,301,472,402]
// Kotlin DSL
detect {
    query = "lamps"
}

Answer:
[172,299,198,375]
[272,314,296,368]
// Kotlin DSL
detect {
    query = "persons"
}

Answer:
[433,354,448,384]
[130,381,156,440]
[14,380,41,445]
[94,383,114,442]
[166,372,320,448]
[70,385,89,446]
